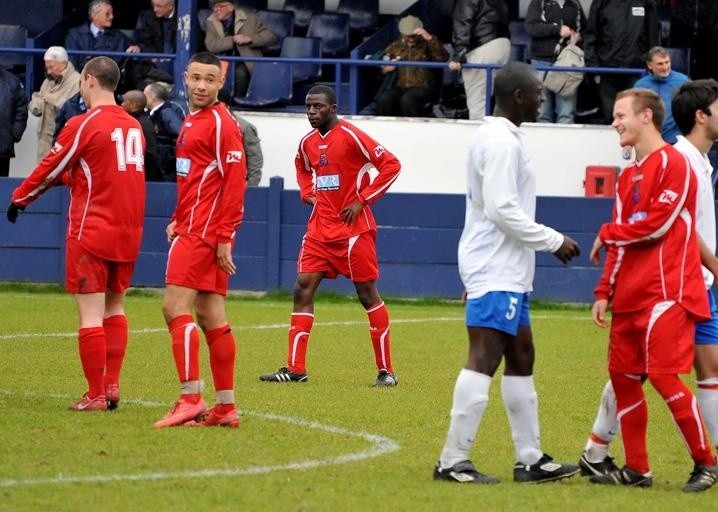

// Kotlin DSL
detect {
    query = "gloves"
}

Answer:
[7,202,25,223]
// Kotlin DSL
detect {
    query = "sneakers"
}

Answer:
[589,465,653,485]
[580,452,620,476]
[154,400,240,428]
[685,454,718,492]
[68,384,120,411]
[513,454,581,482]
[372,369,398,387]
[259,367,307,382]
[434,459,498,483]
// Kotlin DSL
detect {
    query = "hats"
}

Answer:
[398,15,424,36]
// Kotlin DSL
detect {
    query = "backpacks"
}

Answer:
[542,30,585,101]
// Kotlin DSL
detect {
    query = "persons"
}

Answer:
[145,52,250,431]
[432,61,583,487]
[5,56,150,412]
[0,0,717,190]
[256,85,402,388]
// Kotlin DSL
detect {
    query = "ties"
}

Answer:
[95,31,103,42]
